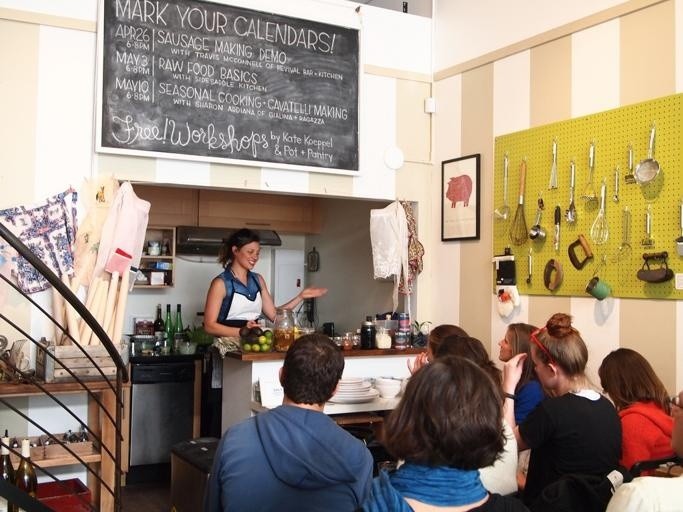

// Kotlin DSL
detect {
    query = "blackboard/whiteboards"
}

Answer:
[95,0,363,177]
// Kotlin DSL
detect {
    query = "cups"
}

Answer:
[375,320,398,336]
[394,329,411,349]
[505,246,510,255]
[583,276,609,301]
[399,313,410,331]
[293,328,315,341]
[141,239,170,257]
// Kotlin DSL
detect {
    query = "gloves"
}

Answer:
[492,254,521,317]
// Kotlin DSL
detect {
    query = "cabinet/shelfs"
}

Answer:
[125,183,198,291]
[0,377,119,512]
[198,189,320,235]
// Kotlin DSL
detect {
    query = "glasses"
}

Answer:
[664,394,682,418]
[529,326,558,363]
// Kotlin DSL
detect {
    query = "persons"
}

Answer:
[201,334,373,512]
[203,227,328,435]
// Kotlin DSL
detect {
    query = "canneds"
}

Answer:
[393,313,412,351]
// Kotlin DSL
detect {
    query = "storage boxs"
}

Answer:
[35,476,91,512]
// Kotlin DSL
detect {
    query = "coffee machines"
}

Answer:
[491,254,521,308]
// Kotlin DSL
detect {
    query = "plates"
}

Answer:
[325,377,380,404]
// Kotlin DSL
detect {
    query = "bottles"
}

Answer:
[374,326,392,349]
[12,438,39,512]
[360,315,373,348]
[338,328,360,349]
[127,303,185,356]
[322,322,334,341]
[0,435,14,511]
[272,308,294,351]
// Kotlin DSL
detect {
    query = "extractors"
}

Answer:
[177,225,282,248]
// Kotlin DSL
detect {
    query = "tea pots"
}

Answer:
[187,311,213,346]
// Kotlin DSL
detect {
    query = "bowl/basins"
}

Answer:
[237,336,275,353]
[375,379,401,399]
[176,342,198,356]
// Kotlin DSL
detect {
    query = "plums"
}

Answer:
[239,326,273,352]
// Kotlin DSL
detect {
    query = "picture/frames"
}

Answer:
[438,153,481,244]
